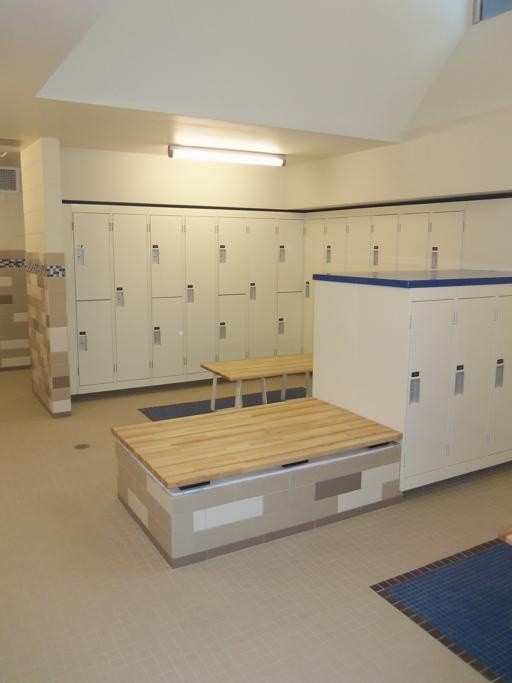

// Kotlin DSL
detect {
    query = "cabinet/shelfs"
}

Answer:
[311,269,512,497]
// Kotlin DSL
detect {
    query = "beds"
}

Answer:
[111,395,406,568]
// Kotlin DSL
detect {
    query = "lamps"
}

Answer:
[167,143,289,169]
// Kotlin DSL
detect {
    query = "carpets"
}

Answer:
[138,386,308,422]
[369,535,512,683]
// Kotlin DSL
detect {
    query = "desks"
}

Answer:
[200,354,315,411]
